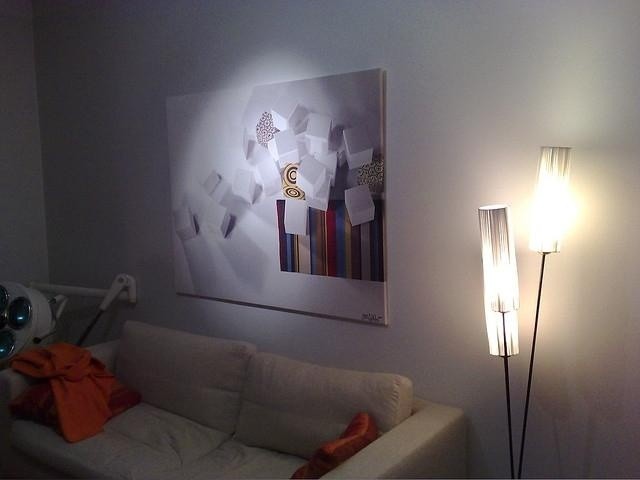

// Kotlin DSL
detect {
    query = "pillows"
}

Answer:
[289,410,379,479]
[8,371,136,438]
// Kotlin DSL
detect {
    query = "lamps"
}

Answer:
[476,145,574,475]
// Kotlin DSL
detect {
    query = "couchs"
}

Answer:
[3,320,469,480]
